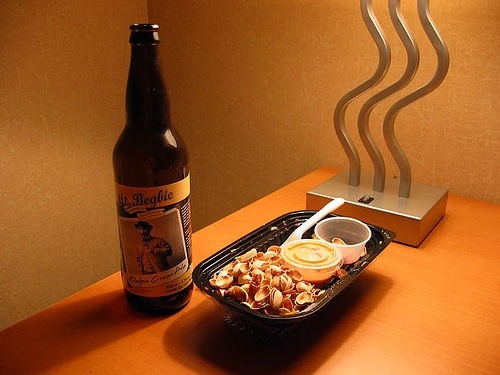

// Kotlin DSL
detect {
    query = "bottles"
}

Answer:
[111,23,195,315]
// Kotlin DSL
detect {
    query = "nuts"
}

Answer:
[209,233,367,318]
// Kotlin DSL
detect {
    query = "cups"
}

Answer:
[281,239,344,290]
[313,216,371,264]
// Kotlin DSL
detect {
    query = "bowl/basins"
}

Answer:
[192,209,396,333]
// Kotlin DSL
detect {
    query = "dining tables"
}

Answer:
[0,162,500,375]
